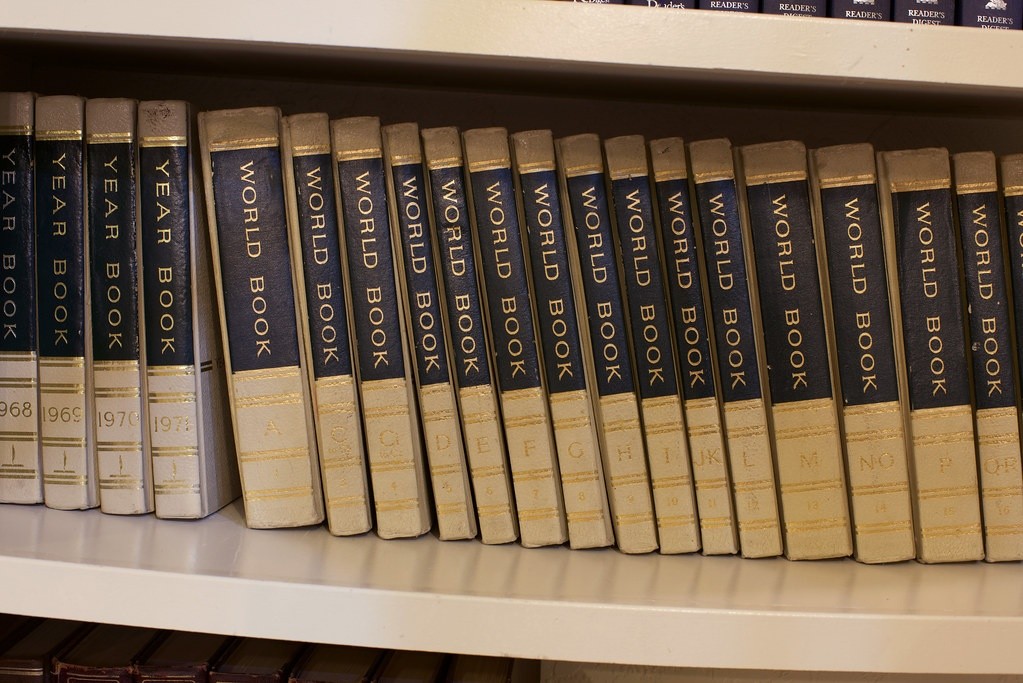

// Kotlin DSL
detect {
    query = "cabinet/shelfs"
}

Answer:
[0,0,1023,682]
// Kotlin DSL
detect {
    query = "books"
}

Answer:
[567,0,1023,31]
[0,90,1023,564]
[1,614,543,683]
